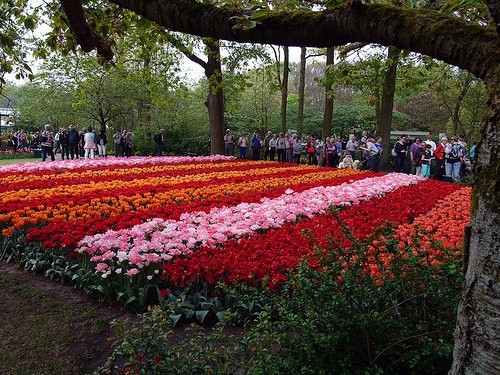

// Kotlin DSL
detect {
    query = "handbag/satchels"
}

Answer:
[94,147,97,151]
[332,150,337,159]
[447,157,461,163]
[307,147,315,152]
[55,134,60,141]
[392,141,399,156]
[40,137,48,142]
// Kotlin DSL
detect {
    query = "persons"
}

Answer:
[250,130,342,168]
[156,129,165,156]
[346,129,478,183]
[113,129,133,157]
[238,132,249,159]
[7,124,108,162]
[224,129,236,156]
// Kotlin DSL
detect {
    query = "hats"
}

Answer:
[226,129,230,132]
[69,124,75,128]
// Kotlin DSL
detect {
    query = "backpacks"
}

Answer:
[100,139,104,145]
[153,135,158,143]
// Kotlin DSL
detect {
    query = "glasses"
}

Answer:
[451,139,455,140]
